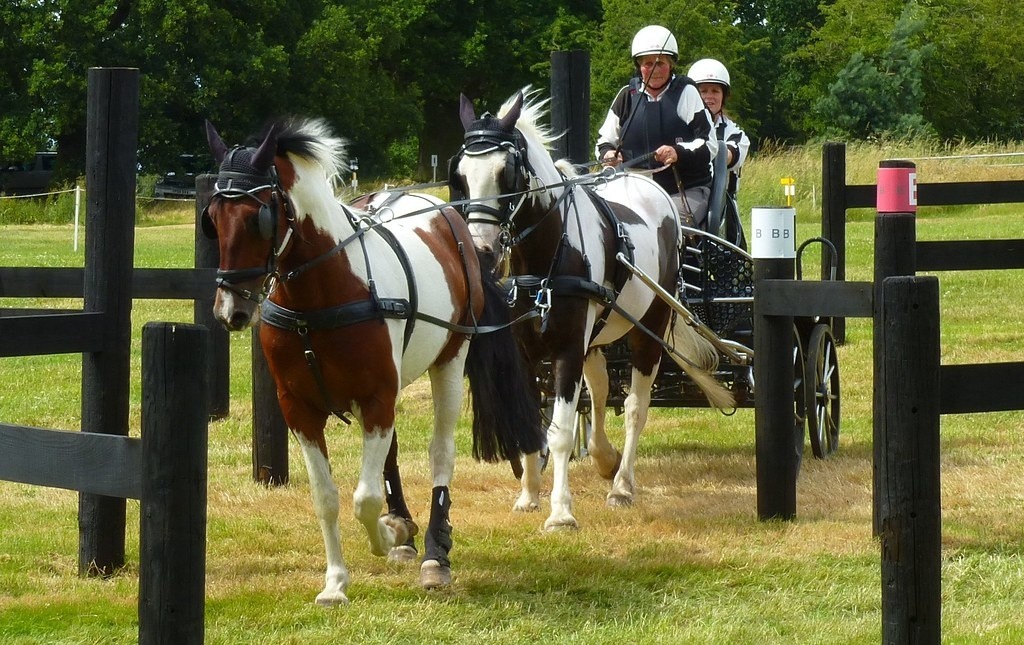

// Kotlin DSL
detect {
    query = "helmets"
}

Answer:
[632,24,678,63]
[687,58,730,88]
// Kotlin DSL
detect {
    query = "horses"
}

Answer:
[202,83,737,609]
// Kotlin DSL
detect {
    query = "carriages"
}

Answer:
[198,81,844,602]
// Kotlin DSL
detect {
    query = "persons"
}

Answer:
[595,25,719,229]
[687,58,751,193]
